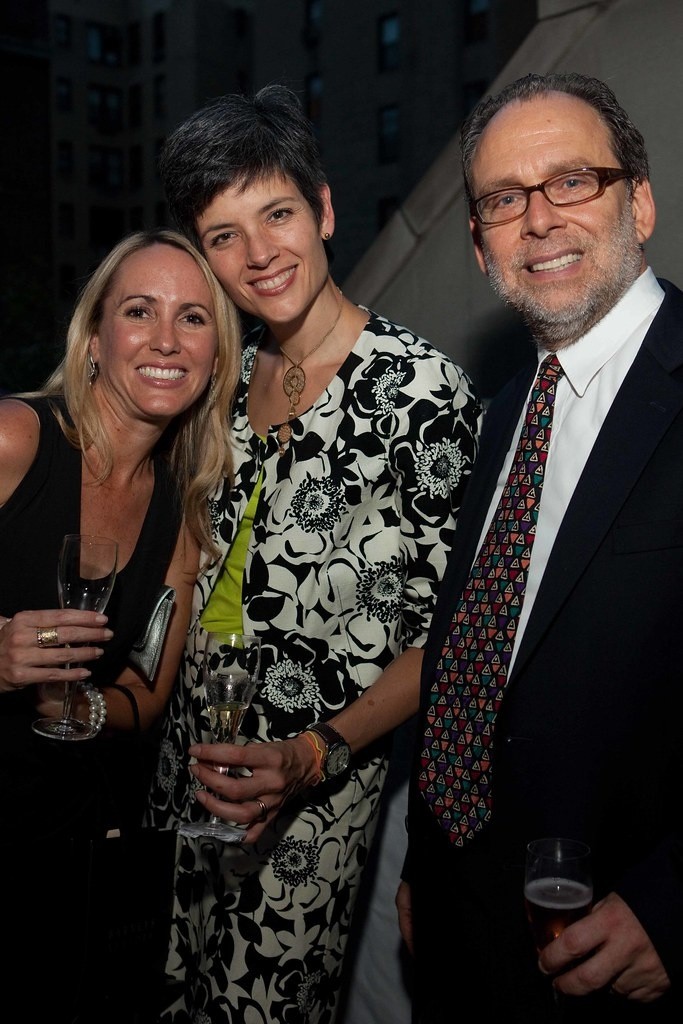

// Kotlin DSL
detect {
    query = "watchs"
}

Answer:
[307,722,352,781]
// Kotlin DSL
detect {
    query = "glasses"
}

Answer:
[470,164,635,223]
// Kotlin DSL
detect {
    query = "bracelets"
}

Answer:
[75,679,108,734]
[297,732,328,788]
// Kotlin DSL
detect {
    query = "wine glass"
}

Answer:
[523,838,597,1023]
[179,629,264,837]
[29,532,121,742]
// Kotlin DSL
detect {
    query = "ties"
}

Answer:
[418,351,565,844]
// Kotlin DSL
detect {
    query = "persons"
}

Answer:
[389,75,683,1024]
[127,86,491,1024]
[1,227,249,1024]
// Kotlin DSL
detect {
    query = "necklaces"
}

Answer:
[276,287,344,459]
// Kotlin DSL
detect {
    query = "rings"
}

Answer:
[38,627,61,649]
[252,797,268,824]
[7,618,14,625]
